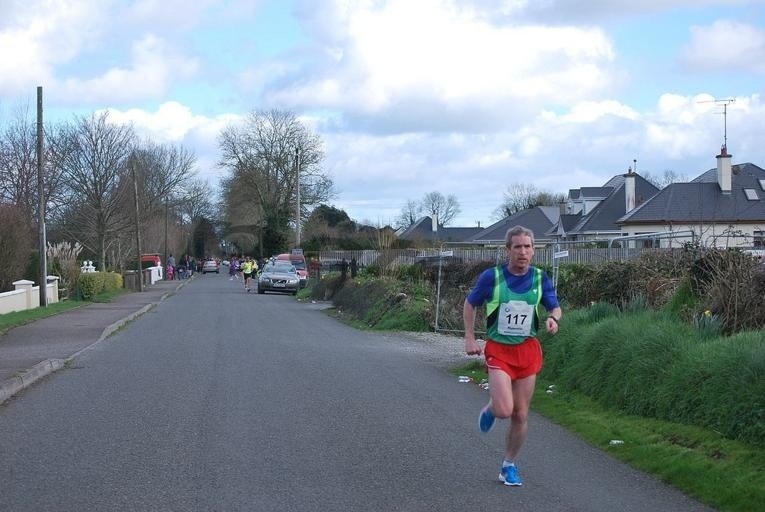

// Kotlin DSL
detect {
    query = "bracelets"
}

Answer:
[550,316,560,326]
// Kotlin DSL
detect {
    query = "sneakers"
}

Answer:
[498,460,523,486]
[479,405,496,434]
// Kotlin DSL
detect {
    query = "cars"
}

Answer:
[202,260,219,274]
[257,260,300,296]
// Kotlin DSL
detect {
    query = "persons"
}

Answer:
[240,256,252,292]
[153,254,258,281]
[463,225,561,486]
[341,258,357,279]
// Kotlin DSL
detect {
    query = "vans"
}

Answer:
[277,253,309,289]
